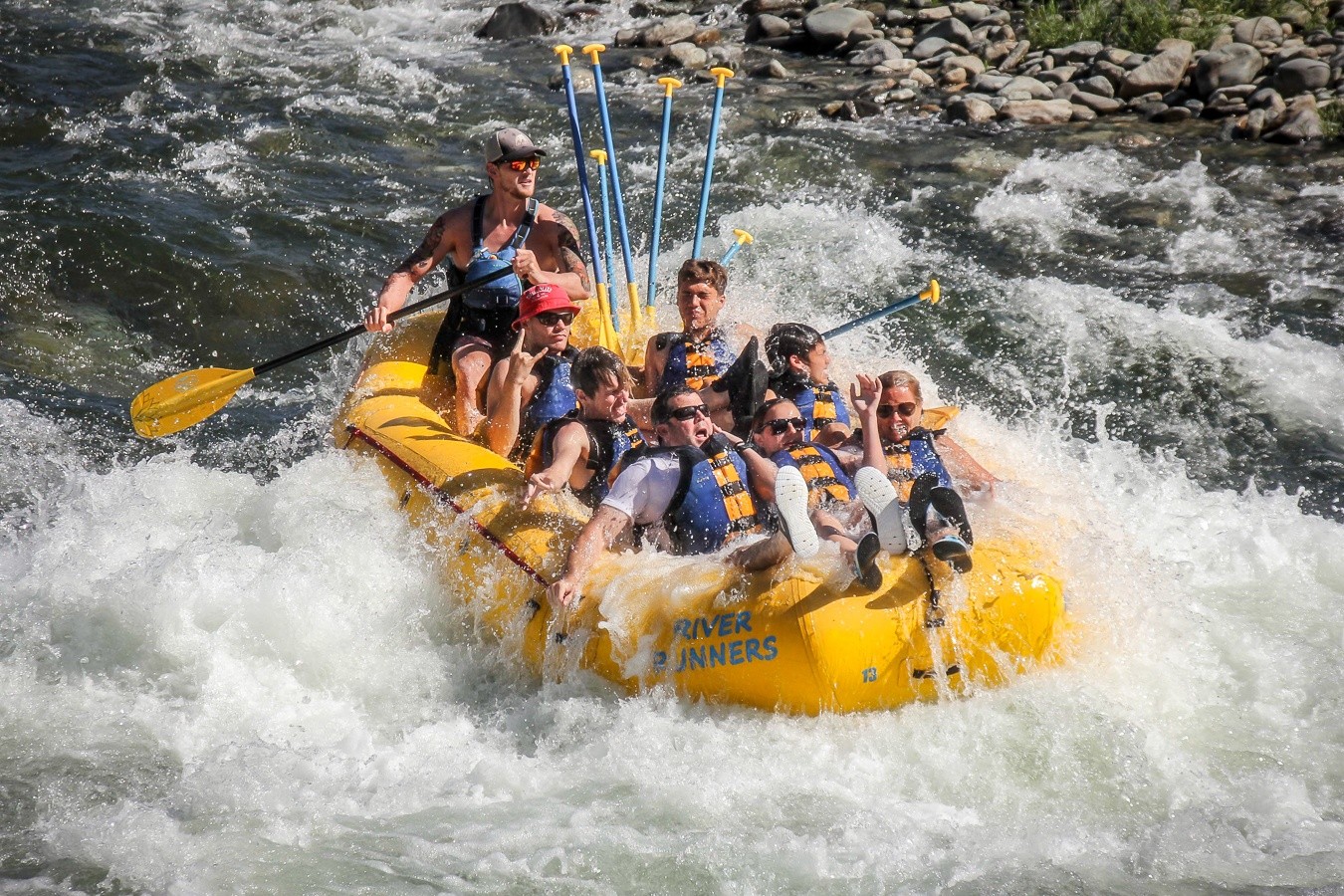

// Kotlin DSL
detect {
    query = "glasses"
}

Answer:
[877,402,916,420]
[510,155,539,173]
[759,416,805,436]
[665,404,712,422]
[536,311,573,326]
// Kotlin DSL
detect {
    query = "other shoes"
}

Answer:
[934,535,975,574]
[853,465,907,556]
[853,532,884,591]
[776,466,820,559]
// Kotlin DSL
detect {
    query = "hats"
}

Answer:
[511,283,580,333]
[487,127,546,165]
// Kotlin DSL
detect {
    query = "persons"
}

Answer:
[364,127,591,438]
[486,258,1004,616]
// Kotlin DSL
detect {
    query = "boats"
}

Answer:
[329,296,1083,721]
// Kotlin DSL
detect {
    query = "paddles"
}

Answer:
[582,43,647,365]
[130,265,529,439]
[553,44,626,364]
[589,150,625,352]
[692,67,734,260]
[818,279,939,341]
[719,229,754,268]
[646,78,682,339]
